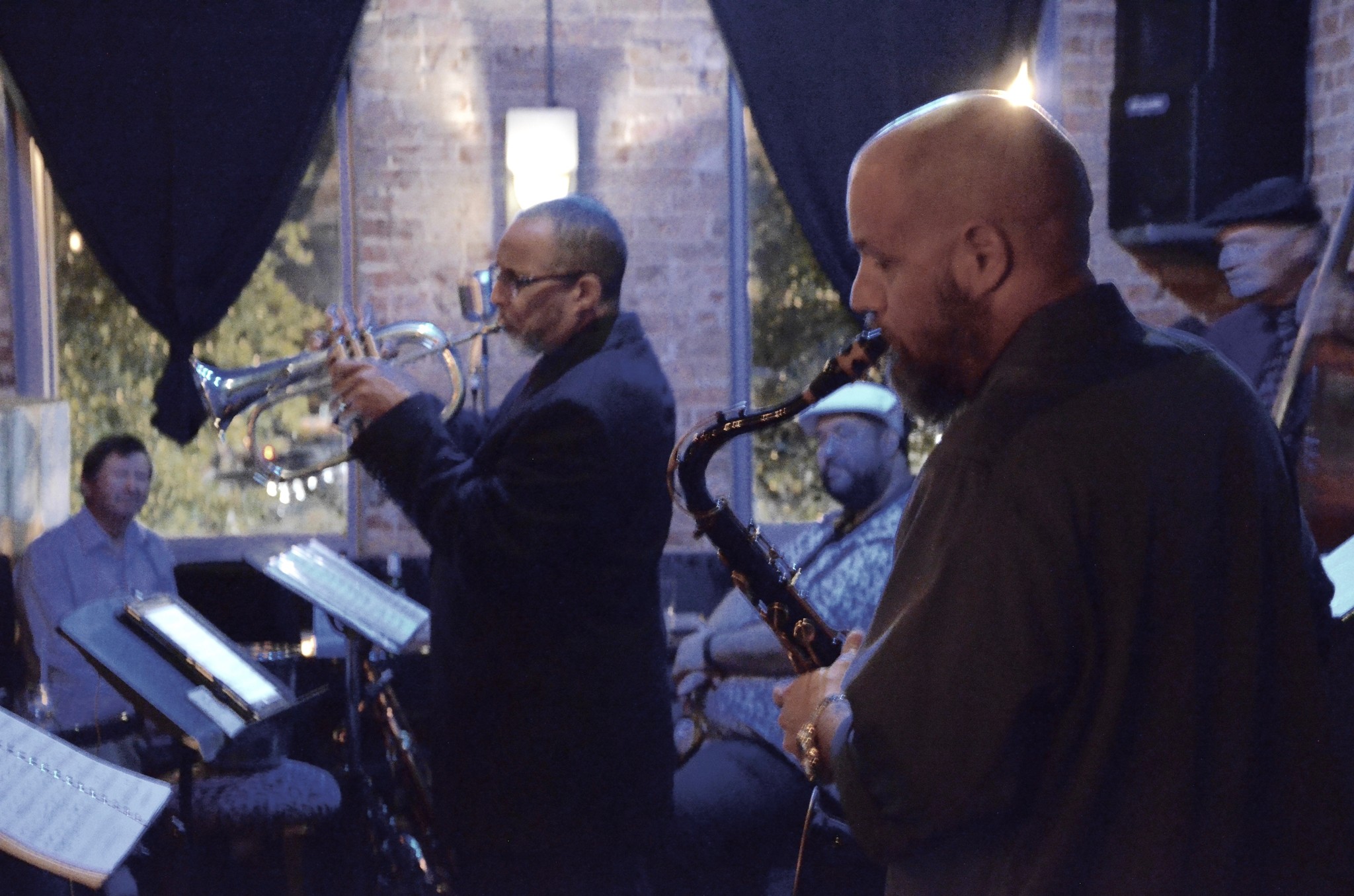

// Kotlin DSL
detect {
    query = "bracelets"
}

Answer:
[797,695,851,787]
[702,633,718,670]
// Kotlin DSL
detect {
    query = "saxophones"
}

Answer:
[664,328,888,680]
[361,621,455,896]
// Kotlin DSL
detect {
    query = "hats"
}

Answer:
[795,380,906,440]
[1199,175,1321,228]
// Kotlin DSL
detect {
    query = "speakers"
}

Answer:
[1105,0,1313,234]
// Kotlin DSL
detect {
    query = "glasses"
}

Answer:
[474,263,583,304]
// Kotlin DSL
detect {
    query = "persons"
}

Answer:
[673,380,918,896]
[772,90,1354,896]
[329,194,679,896]
[1201,176,1354,435]
[12,433,181,770]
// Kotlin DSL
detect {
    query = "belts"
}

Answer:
[57,711,131,748]
